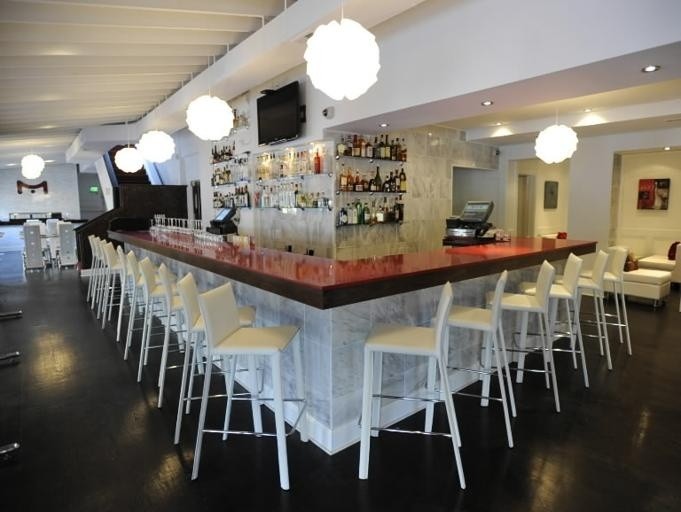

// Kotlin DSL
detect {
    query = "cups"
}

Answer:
[496,229,512,242]
[207,232,256,257]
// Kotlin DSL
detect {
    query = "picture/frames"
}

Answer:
[543,181,558,209]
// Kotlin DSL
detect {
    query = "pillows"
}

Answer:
[667,242,680,260]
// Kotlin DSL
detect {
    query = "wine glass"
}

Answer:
[149,215,206,243]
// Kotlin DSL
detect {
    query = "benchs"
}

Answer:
[606,269,671,309]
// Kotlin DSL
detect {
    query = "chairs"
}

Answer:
[637,243,680,286]
[8,211,77,272]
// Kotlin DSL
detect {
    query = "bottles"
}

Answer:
[210,140,325,188]
[336,160,408,194]
[334,194,406,229]
[212,182,333,210]
[337,131,408,162]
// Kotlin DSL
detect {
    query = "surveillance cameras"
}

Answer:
[323,107,334,118]
[493,148,500,156]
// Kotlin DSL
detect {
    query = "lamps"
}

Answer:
[181,52,232,139]
[302,3,381,106]
[113,119,144,173]
[135,100,175,164]
[532,107,580,166]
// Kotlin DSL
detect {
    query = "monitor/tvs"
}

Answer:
[257,81,300,145]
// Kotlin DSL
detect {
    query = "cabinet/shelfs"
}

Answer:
[210,142,332,211]
[330,141,406,228]
